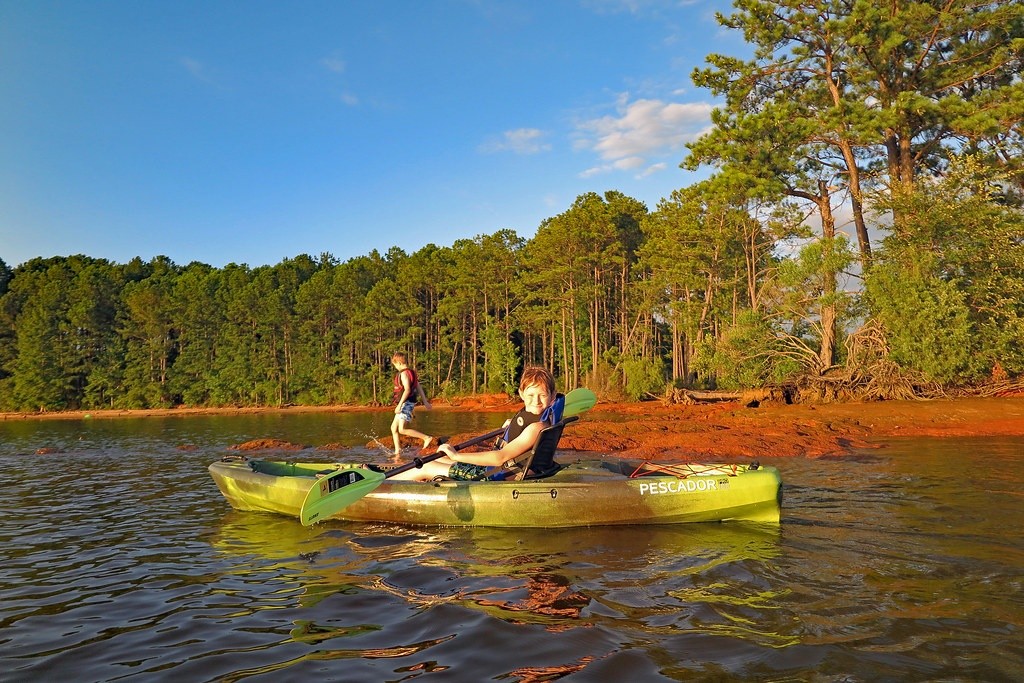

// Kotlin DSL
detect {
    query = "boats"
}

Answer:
[206,448,785,533]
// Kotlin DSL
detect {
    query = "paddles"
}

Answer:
[298,385,598,529]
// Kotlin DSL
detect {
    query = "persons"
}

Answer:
[386,352,433,461]
[361,365,558,482]
